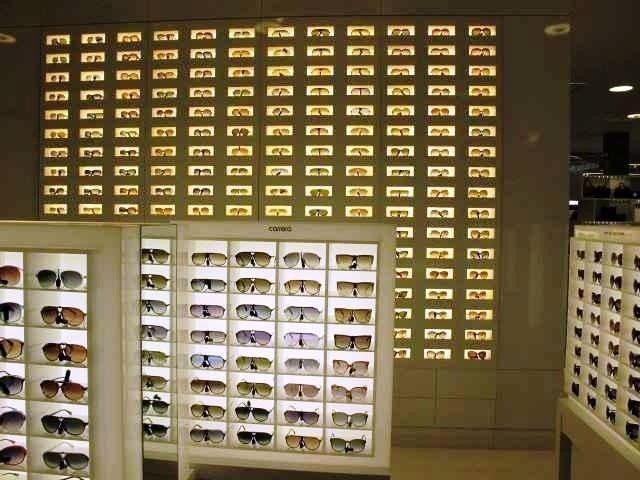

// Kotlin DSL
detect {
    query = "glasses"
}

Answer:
[142,423,170,437]
[141,274,171,290]
[235,402,274,422]
[49,28,491,219]
[237,425,274,446]
[285,358,319,372]
[190,304,225,319]
[337,281,374,297]
[190,354,226,370]
[140,300,170,315]
[393,231,489,360]
[284,332,324,348]
[334,334,372,350]
[236,356,273,370]
[572,250,640,440]
[191,279,227,292]
[142,400,170,415]
[237,382,273,397]
[286,428,321,450]
[285,280,321,296]
[235,252,275,267]
[332,409,368,427]
[236,304,275,320]
[331,385,367,402]
[141,325,169,340]
[142,350,170,365]
[336,254,373,270]
[190,404,226,419]
[0,266,88,480]
[284,383,320,399]
[141,249,172,264]
[192,253,227,266]
[284,405,319,425]
[284,252,321,269]
[236,278,274,294]
[284,306,323,321]
[335,308,372,324]
[236,330,271,345]
[333,360,369,377]
[330,432,366,453]
[190,330,226,344]
[190,424,225,444]
[141,376,169,391]
[190,379,226,394]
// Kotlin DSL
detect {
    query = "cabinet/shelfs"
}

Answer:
[544,219,640,479]
[5,214,189,480]
[167,216,400,477]
[33,21,502,436]
